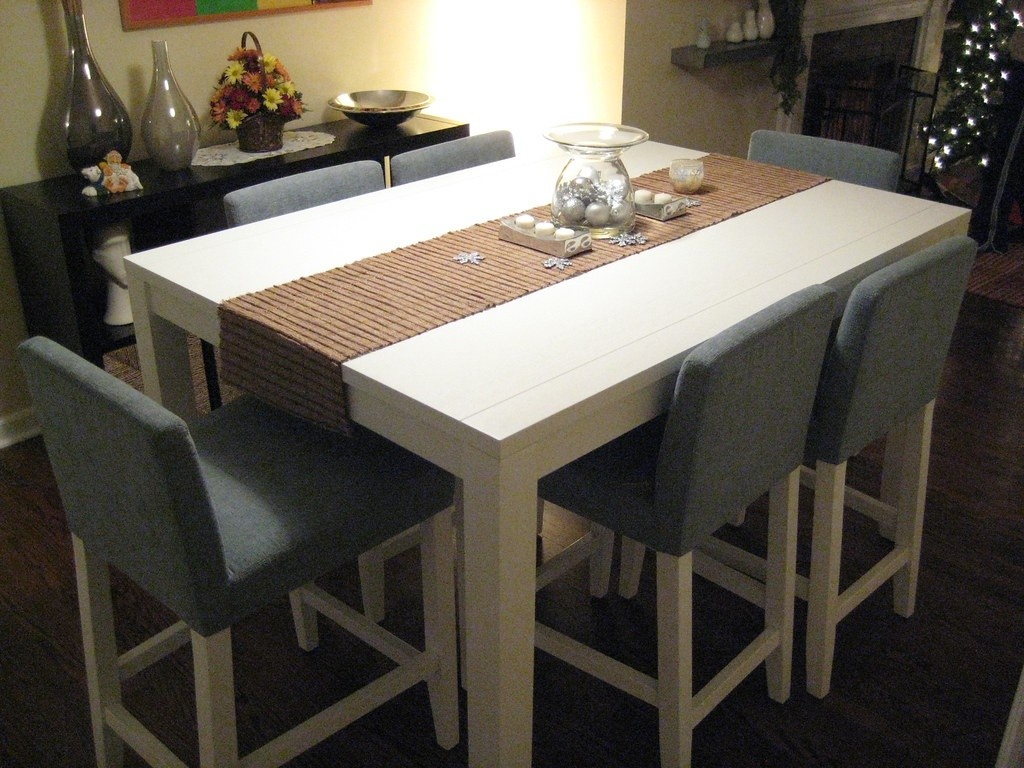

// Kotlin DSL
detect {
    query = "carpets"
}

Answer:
[1,289,1023,768]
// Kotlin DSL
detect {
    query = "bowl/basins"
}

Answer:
[327,90,433,128]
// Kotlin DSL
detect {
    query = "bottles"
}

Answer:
[51,1,134,175]
[697,23,710,49]
[726,0,775,44]
[141,39,201,169]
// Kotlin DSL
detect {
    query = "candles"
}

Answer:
[654,193,672,204]
[535,220,555,236]
[515,212,536,229]
[635,189,652,202]
[554,227,575,239]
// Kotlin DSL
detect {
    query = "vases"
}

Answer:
[141,40,201,168]
[236,114,301,153]
[56,0,136,176]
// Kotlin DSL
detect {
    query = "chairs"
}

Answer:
[221,160,427,624]
[0,114,471,370]
[617,236,980,700]
[14,335,460,767]
[391,130,516,186]
[535,282,839,768]
[746,130,901,191]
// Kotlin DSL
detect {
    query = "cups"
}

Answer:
[668,159,703,194]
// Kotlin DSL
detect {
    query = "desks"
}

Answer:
[124,131,972,768]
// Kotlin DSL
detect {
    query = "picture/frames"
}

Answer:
[118,0,371,32]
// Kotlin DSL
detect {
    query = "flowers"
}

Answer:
[205,46,313,131]
